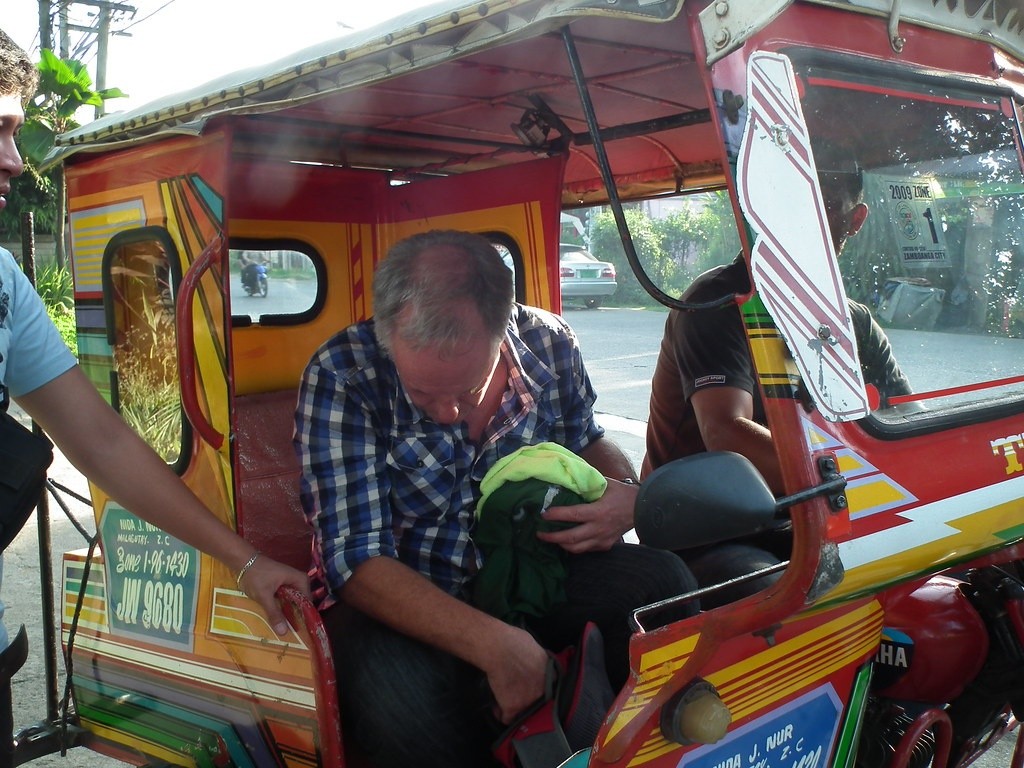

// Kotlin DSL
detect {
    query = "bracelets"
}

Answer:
[621,477,641,486]
[237,552,260,584]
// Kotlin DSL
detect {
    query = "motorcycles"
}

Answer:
[50,1,1024,768]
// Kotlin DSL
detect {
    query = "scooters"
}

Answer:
[239,258,271,299]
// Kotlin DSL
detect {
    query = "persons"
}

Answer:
[639,167,931,609]
[0,28,312,768]
[238,250,271,292]
[293,228,701,768]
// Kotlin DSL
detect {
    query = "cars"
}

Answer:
[494,241,619,309]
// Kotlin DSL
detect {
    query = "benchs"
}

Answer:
[229,389,345,768]
